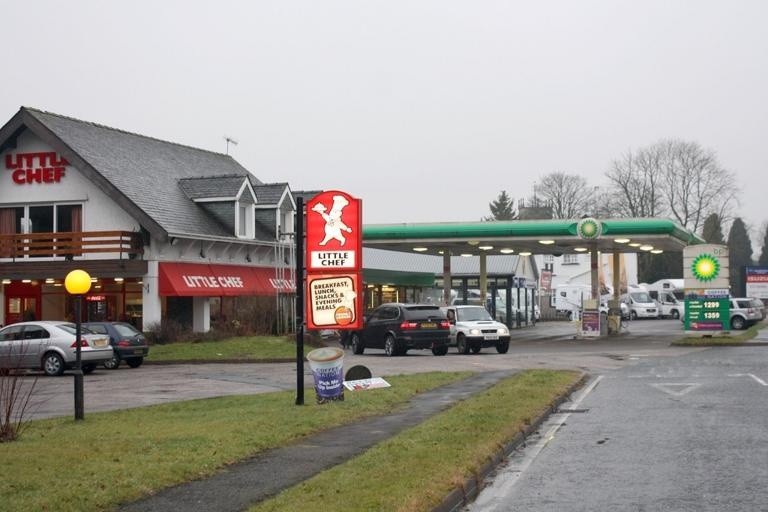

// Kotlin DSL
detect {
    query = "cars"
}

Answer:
[75,321,150,370]
[0,320,114,377]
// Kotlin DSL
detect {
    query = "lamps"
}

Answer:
[499,248,514,254]
[91,277,98,283]
[53,280,62,287]
[45,278,56,284]
[21,279,32,284]
[169,237,179,247]
[284,248,290,265]
[138,281,149,294]
[200,240,207,258]
[1,279,11,284]
[640,244,654,251]
[246,245,252,262]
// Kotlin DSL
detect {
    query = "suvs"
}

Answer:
[728,296,767,331]
[349,287,541,357]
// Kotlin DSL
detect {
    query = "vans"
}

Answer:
[555,278,685,331]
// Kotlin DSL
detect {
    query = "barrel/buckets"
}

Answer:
[306,347,344,397]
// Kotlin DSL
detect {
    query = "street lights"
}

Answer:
[64,269,91,421]
